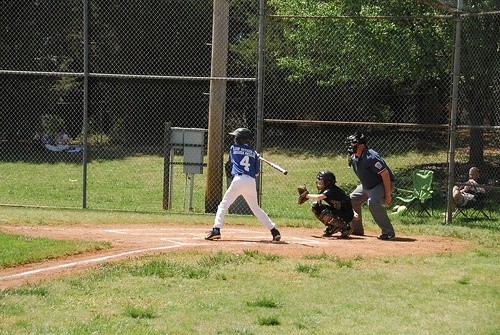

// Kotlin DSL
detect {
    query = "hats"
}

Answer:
[228,128,255,144]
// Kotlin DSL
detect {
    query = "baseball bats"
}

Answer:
[259,156,288,175]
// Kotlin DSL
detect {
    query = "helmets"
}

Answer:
[316,169,337,189]
[345,132,367,151]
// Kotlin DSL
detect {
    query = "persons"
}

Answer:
[345,131,396,240]
[453,167,487,208]
[205,127,281,242]
[302,171,360,239]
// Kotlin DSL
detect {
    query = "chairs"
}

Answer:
[456,177,490,220]
[394,169,435,219]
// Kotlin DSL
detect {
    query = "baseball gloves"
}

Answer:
[297,186,309,205]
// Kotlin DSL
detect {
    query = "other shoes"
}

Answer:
[441,221,446,224]
[350,231,364,236]
[338,225,353,239]
[205,228,221,241]
[271,230,281,243]
[377,232,394,240]
[325,225,340,235]
